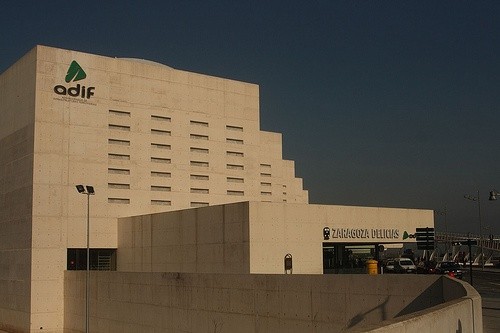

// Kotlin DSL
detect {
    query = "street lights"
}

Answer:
[488,190,499,201]
[75,183,94,333]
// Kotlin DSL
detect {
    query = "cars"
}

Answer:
[385,261,396,272]
[433,260,462,280]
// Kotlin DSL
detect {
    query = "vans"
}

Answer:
[393,258,417,274]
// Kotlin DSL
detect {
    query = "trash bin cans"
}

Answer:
[365,260,378,275]
